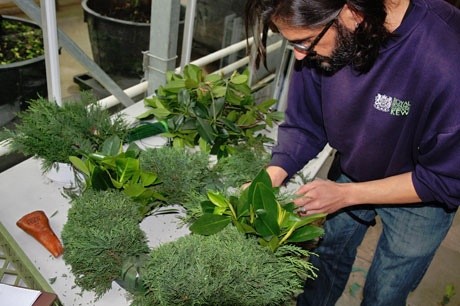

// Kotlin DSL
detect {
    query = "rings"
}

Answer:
[300,206,307,214]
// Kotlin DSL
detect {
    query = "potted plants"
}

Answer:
[0,15,47,104]
[81,0,186,79]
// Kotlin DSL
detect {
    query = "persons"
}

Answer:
[240,0,460,306]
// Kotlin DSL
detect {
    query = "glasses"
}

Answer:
[286,19,335,55]
